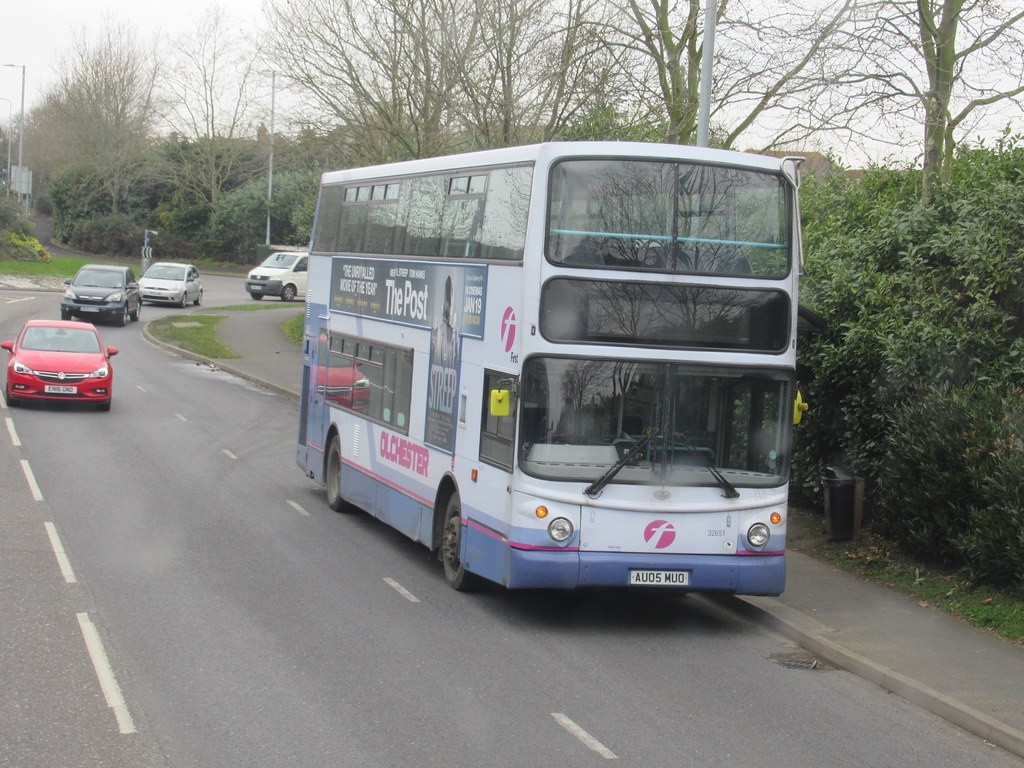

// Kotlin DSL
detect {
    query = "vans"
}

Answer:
[245,252,308,302]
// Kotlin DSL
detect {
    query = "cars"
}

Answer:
[1,319,119,412]
[61,264,142,327]
[138,261,204,308]
[317,334,369,412]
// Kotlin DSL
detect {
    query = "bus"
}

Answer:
[295,140,808,599]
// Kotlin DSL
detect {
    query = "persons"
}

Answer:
[429,273,459,370]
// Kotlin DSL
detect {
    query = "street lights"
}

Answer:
[0,98,12,197]
[2,64,25,202]
